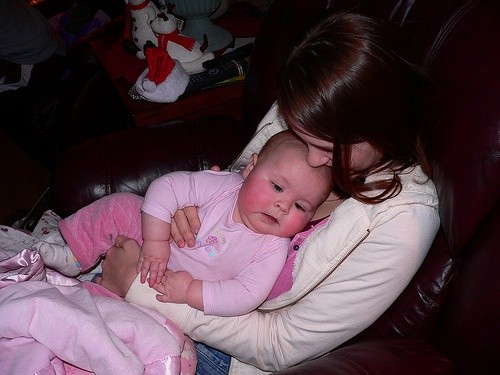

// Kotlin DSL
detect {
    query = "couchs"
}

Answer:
[61,0,500,375]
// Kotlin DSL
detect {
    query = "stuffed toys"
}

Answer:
[125,0,208,62]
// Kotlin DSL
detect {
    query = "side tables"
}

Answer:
[89,0,262,133]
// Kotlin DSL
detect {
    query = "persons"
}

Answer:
[31,130,334,318]
[169,12,440,375]
[1,1,79,168]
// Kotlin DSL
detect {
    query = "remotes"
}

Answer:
[202,42,256,70]
[186,62,240,90]
